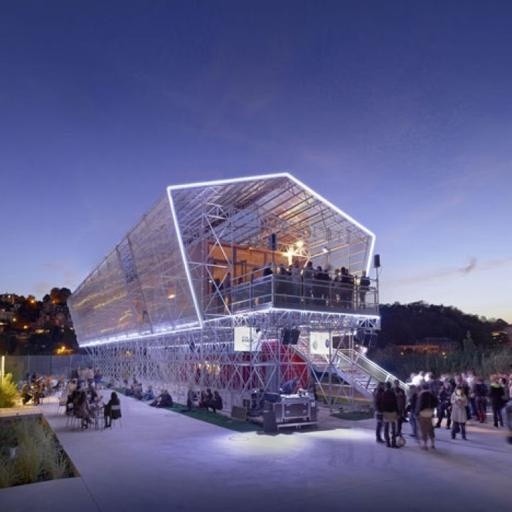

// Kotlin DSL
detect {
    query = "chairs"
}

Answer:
[17,375,121,430]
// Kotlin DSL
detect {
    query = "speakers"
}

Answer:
[268,233,277,250]
[373,254,381,268]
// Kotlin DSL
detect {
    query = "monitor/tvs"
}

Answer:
[282,329,300,344]
[333,336,351,349]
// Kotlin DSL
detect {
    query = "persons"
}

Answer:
[186,387,223,412]
[263,261,370,309]
[373,371,512,449]
[279,376,300,394]
[21,365,121,428]
[121,377,173,408]
